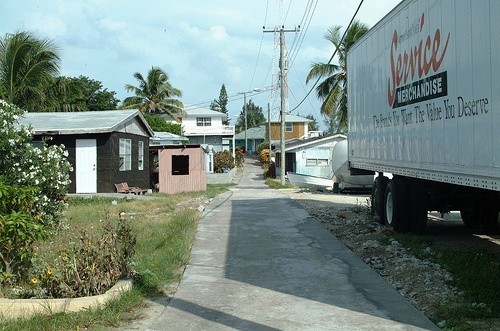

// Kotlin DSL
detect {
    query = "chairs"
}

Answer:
[122,182,147,195]
[114,184,141,195]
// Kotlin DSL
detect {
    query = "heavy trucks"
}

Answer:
[347,0,500,244]
